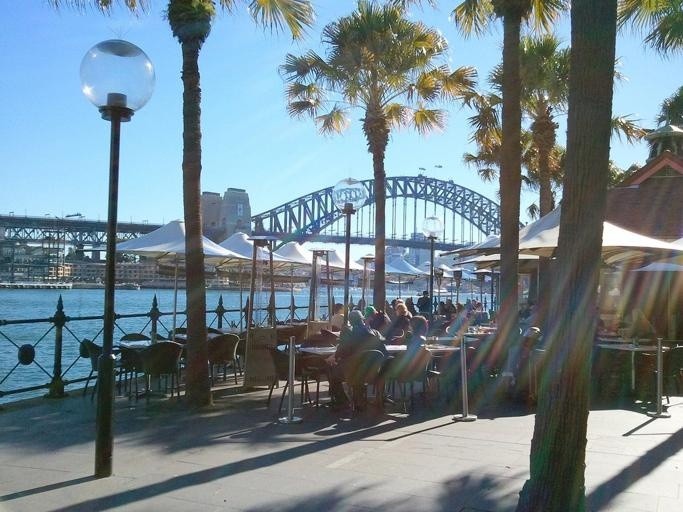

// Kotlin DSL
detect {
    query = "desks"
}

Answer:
[422,260,433,294]
[421,216,442,324]
[75,40,153,481]
[331,176,366,325]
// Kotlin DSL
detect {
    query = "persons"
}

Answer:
[327,291,605,411]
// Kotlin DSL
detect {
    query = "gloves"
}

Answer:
[416,286,457,297]
[114,281,141,290]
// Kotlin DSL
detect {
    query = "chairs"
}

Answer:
[78,290,542,419]
[597,309,680,415]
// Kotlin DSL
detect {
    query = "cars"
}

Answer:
[632,335,640,348]
